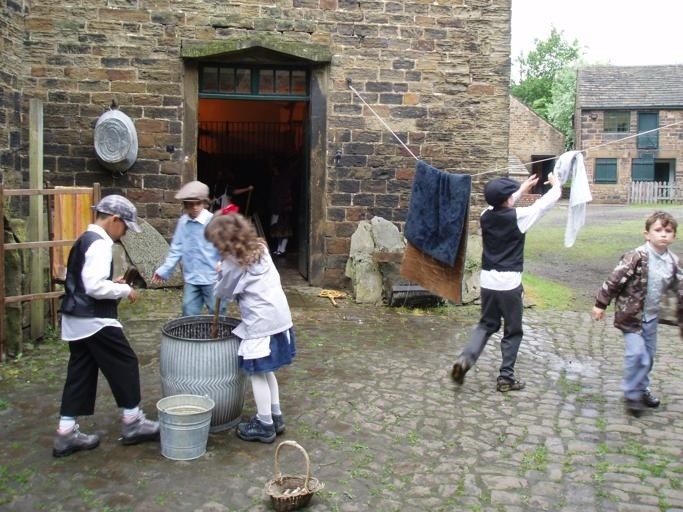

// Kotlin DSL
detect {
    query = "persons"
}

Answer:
[150,179,226,318]
[591,212,682,414]
[273,239,289,258]
[449,172,562,392]
[51,195,161,459]
[204,213,295,443]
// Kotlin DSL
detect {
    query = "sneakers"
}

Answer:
[120,410,160,445]
[52,423,99,458]
[620,393,660,413]
[236,411,285,443]
[451,357,470,387]
[496,376,526,392]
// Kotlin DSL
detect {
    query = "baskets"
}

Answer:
[265,442,326,511]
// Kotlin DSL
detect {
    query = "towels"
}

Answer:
[552,150,592,248]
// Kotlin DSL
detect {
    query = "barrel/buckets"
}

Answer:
[155,394,216,461]
[161,314,245,432]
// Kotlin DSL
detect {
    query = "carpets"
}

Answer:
[398,159,471,305]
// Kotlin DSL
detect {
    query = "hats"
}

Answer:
[174,181,209,202]
[95,195,145,234]
[483,176,520,206]
